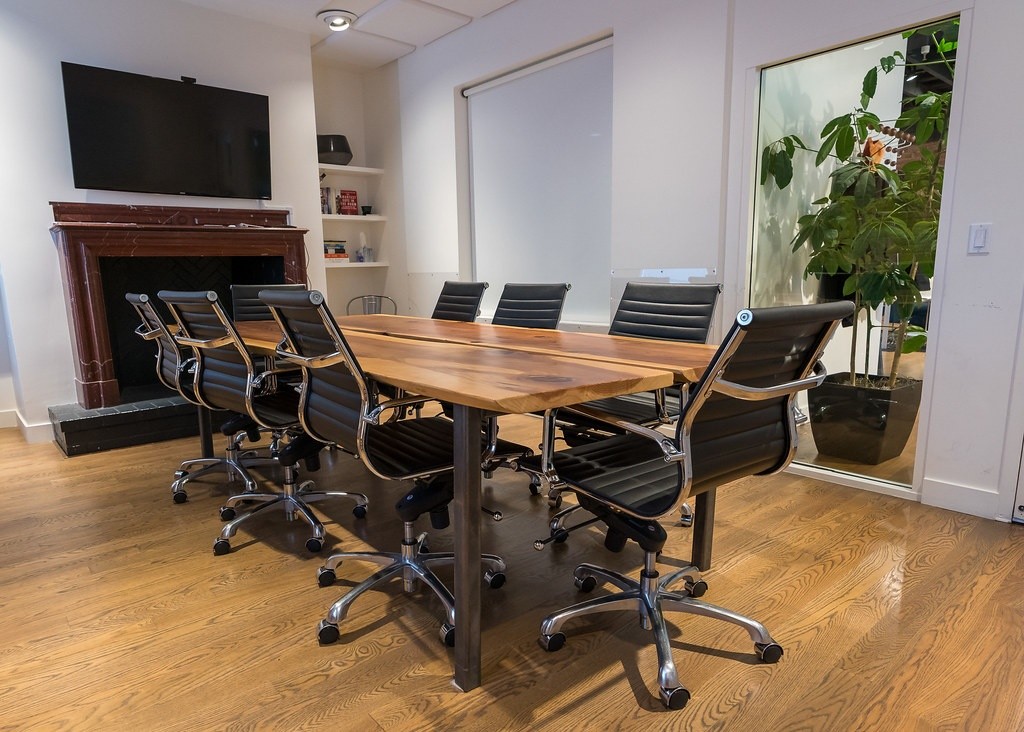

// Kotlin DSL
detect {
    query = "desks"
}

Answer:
[156,312,728,694]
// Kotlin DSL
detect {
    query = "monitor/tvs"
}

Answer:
[60,61,272,201]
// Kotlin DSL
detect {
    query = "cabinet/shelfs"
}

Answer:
[317,161,390,269]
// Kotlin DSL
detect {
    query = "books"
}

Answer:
[324,240,350,263]
[320,186,358,214]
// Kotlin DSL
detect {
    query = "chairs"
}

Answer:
[158,289,369,558]
[258,288,507,645]
[533,276,724,550]
[538,299,857,709]
[360,278,571,435]
[126,293,298,502]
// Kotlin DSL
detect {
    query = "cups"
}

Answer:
[361,206,372,215]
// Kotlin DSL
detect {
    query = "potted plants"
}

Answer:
[761,15,966,470]
